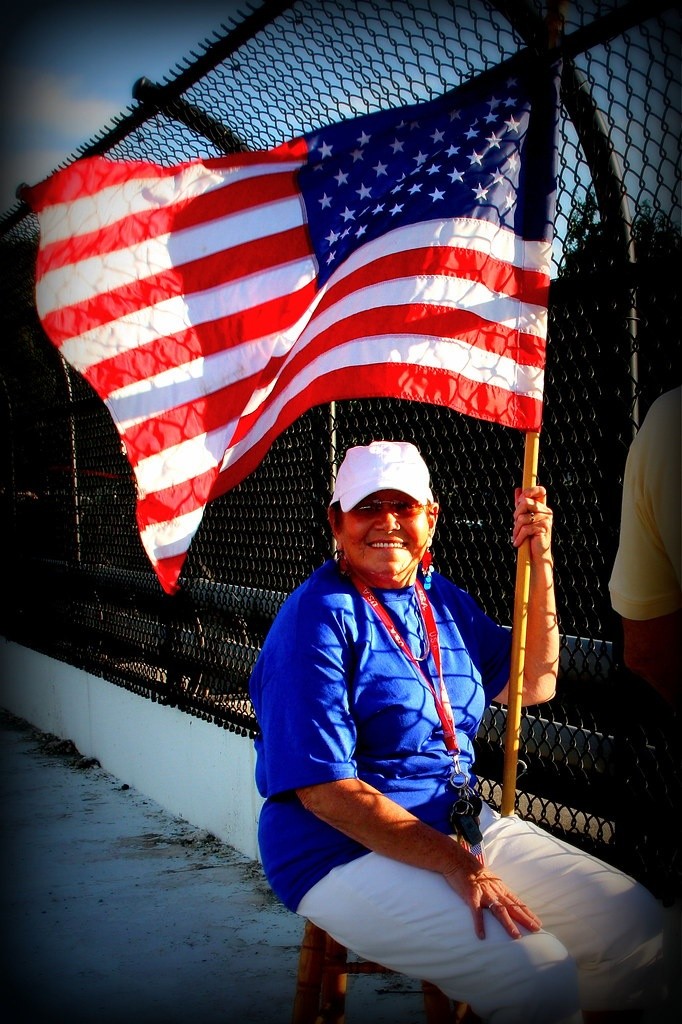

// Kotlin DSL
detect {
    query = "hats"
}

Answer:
[329,441,432,513]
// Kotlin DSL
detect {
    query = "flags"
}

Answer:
[37,37,557,597]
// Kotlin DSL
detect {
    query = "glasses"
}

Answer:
[353,500,421,515]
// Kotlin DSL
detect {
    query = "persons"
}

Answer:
[255,438,658,1013]
[606,244,680,912]
